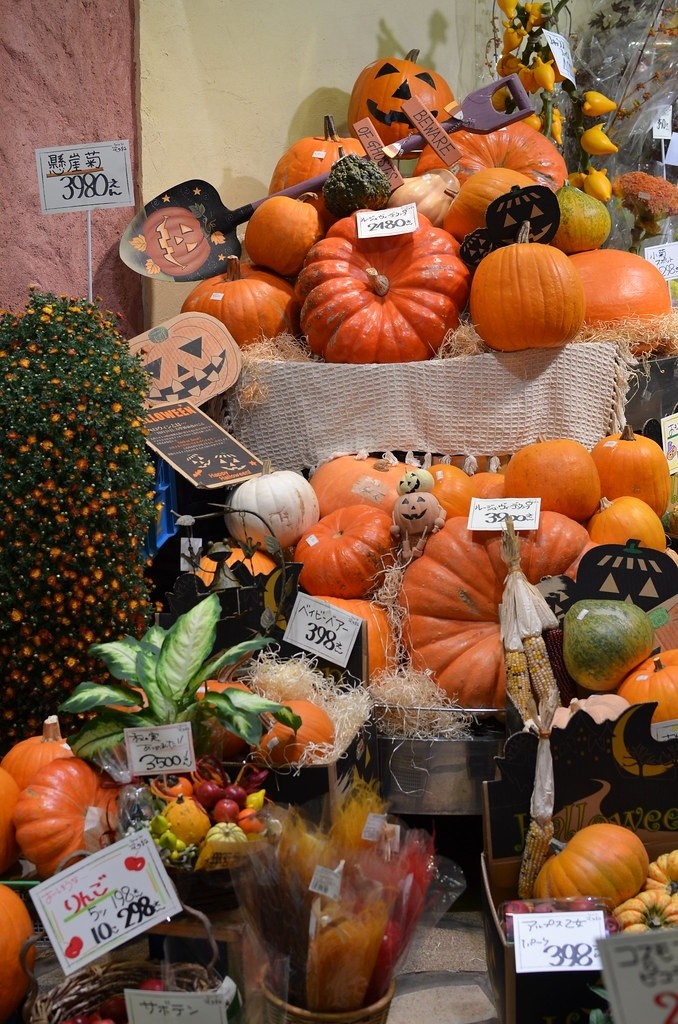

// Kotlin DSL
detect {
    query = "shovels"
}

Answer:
[114,72,536,285]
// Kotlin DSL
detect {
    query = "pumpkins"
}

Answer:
[0,54,678,1019]
